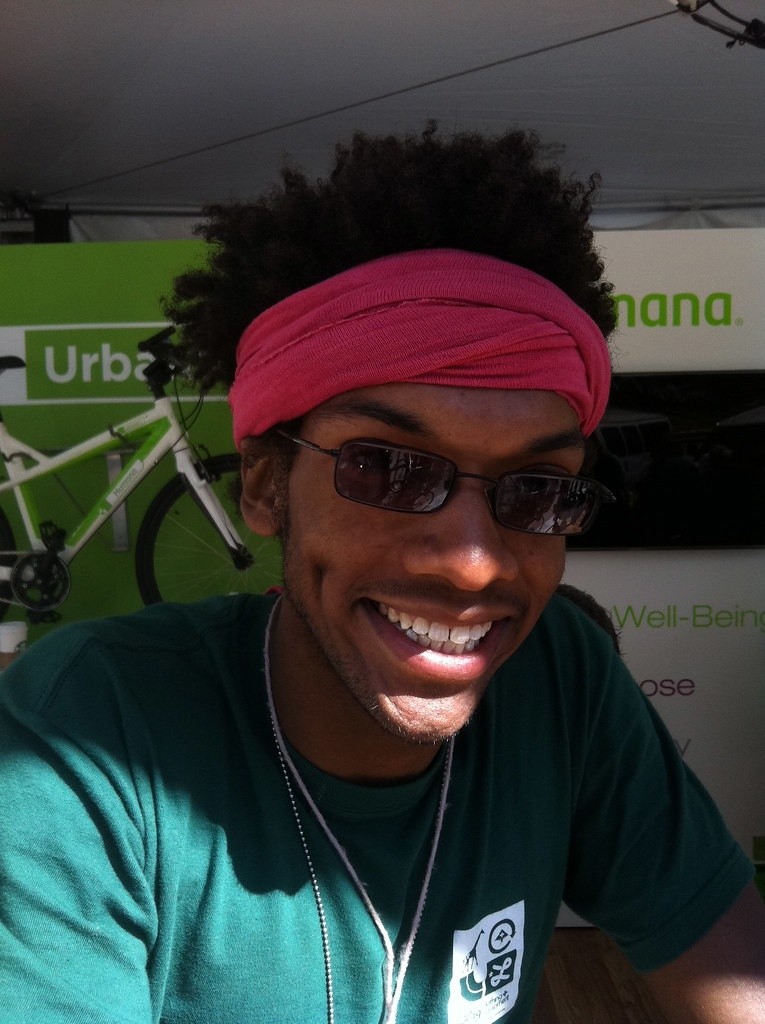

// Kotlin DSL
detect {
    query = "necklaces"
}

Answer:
[262,592,471,1024]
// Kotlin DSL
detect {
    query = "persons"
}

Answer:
[0,126,765,1024]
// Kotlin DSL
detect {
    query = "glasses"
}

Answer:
[270,423,617,539]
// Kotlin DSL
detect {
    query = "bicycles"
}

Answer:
[0,326,284,624]
